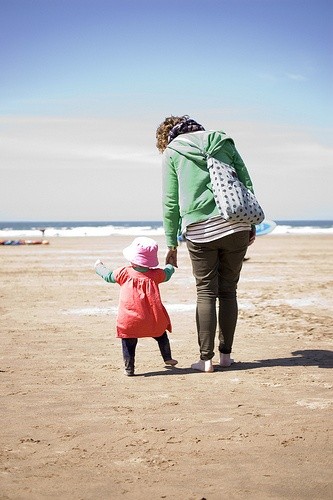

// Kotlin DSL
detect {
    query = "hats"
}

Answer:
[123,237,159,267]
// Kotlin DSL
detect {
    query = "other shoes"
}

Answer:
[124,371,133,375]
[165,360,178,365]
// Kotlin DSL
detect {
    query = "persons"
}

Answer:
[94,237,178,376]
[155,113,256,373]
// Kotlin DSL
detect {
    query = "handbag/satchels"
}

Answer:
[206,155,265,225]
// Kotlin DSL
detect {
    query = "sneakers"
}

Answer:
[191,360,214,372]
[220,353,234,367]
